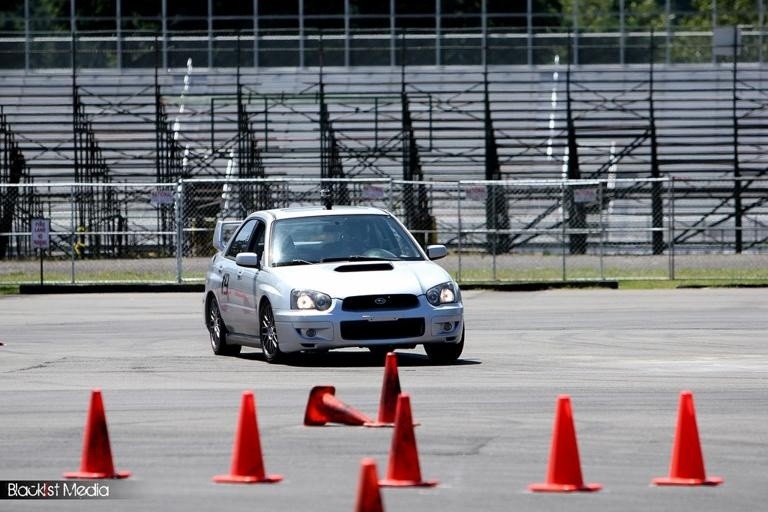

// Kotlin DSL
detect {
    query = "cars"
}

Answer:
[201,196,468,367]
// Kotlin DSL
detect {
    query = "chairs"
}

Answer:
[273,231,297,257]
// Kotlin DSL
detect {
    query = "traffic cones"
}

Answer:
[354,456,387,512]
[61,386,131,479]
[363,351,422,430]
[526,392,603,498]
[649,390,725,488]
[376,393,441,490]
[299,382,376,429]
[211,390,284,485]
